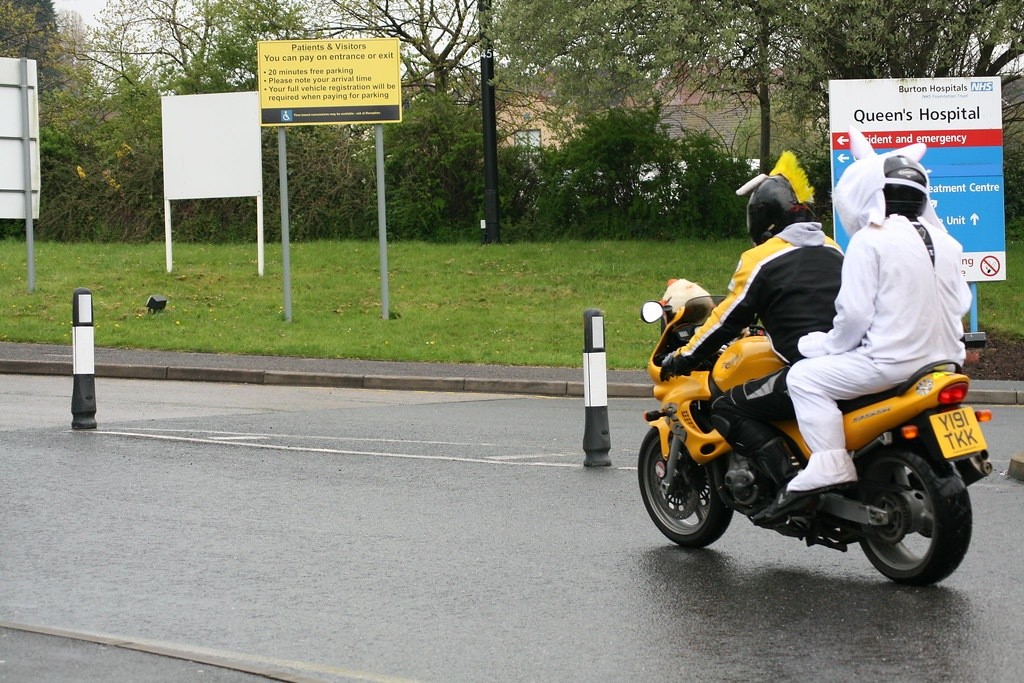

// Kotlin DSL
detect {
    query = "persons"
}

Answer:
[661,174,847,527]
[784,126,974,492]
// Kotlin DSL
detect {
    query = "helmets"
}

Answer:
[735,150,816,246]
[882,155,928,218]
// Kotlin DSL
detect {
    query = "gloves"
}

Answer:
[661,351,683,381]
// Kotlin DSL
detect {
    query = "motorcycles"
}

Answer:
[638,277,993,589]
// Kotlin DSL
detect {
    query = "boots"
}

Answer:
[745,436,822,525]
